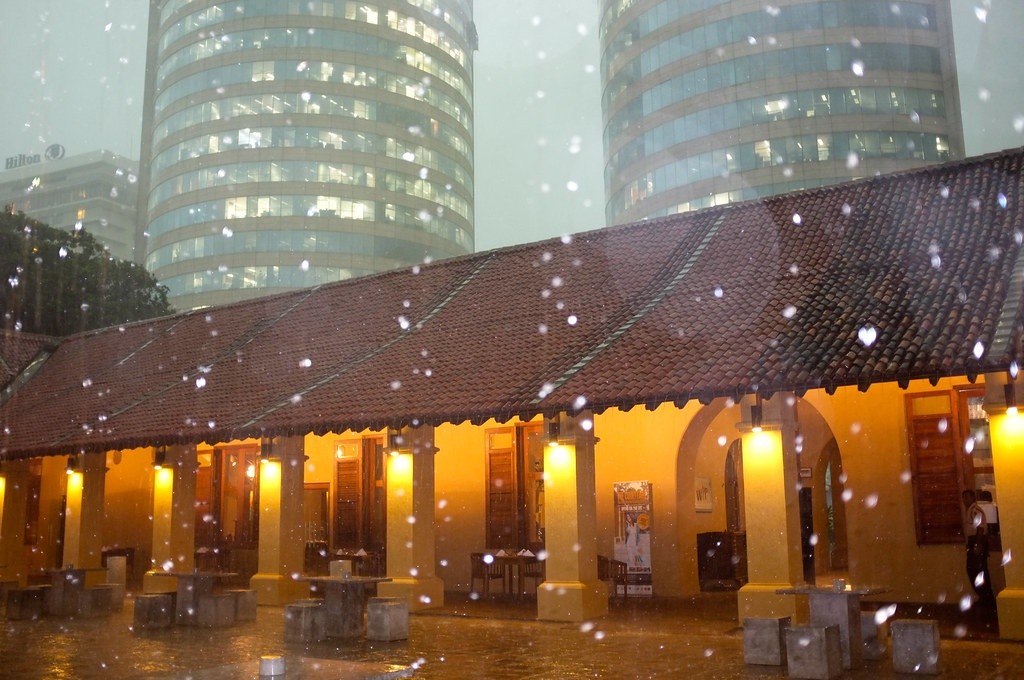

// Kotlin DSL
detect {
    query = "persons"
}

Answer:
[961,488,1000,610]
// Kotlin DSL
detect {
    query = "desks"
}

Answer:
[481,555,539,603]
[154,570,238,628]
[194,551,214,571]
[27,566,110,618]
[773,585,894,670]
[299,575,393,639]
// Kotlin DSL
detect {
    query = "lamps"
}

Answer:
[65,458,75,474]
[548,422,559,446]
[750,405,763,432]
[1003,384,1019,417]
[153,451,164,470]
[388,434,399,456]
[260,444,270,463]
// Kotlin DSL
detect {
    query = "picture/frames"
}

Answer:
[694,475,713,512]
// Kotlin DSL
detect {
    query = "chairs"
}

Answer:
[517,557,545,597]
[597,555,627,600]
[470,552,506,599]
[215,548,233,584]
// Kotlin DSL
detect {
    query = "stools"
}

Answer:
[0,581,943,680]
